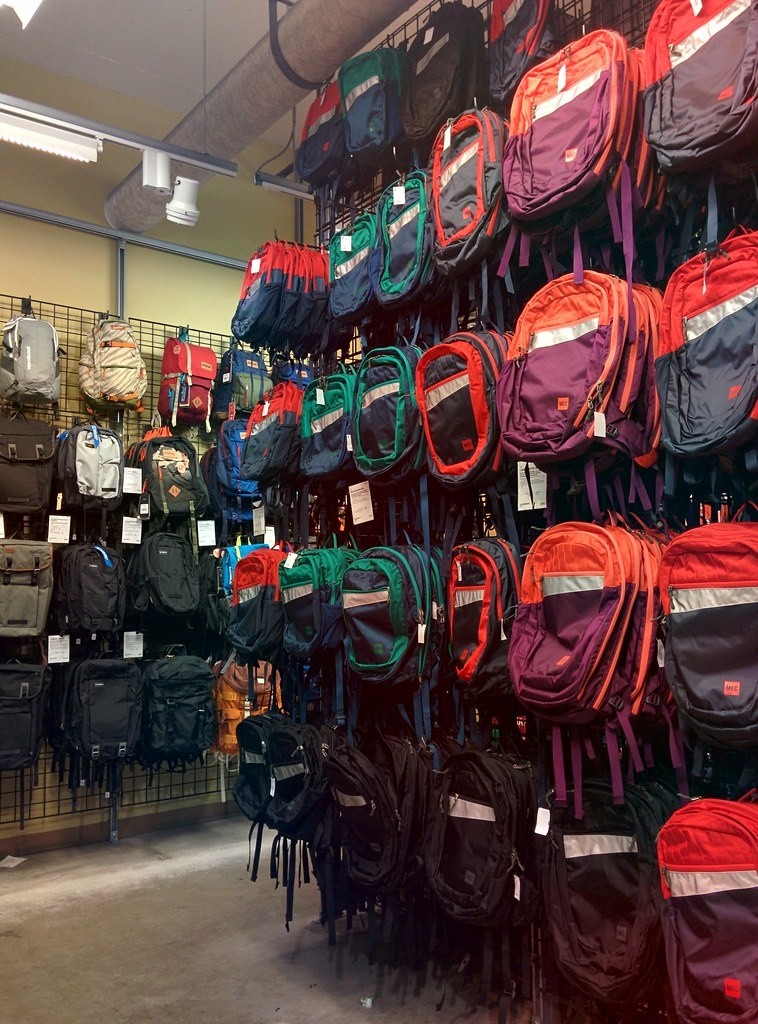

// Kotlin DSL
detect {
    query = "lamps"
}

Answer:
[141,150,200,227]
[253,171,314,201]
[0,103,103,163]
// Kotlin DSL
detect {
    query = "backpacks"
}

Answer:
[220,2,758,1023]
[0,299,222,829]
